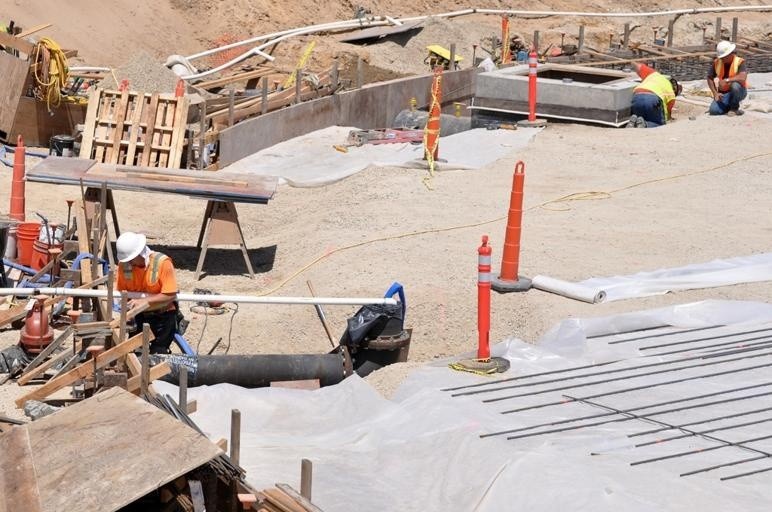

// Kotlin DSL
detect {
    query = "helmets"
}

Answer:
[716,40,736,59]
[669,78,682,95]
[116,232,146,263]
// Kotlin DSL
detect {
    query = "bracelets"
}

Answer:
[725,79,729,82]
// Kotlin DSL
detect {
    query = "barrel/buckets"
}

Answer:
[15,222,43,266]
[30,238,64,276]
[48,134,74,157]
[38,223,67,247]
[4,227,18,258]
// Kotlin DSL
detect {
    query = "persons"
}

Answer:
[115,232,177,353]
[626,60,682,128]
[706,40,747,115]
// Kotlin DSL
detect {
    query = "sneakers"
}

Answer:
[728,108,744,116]
[626,114,645,128]
